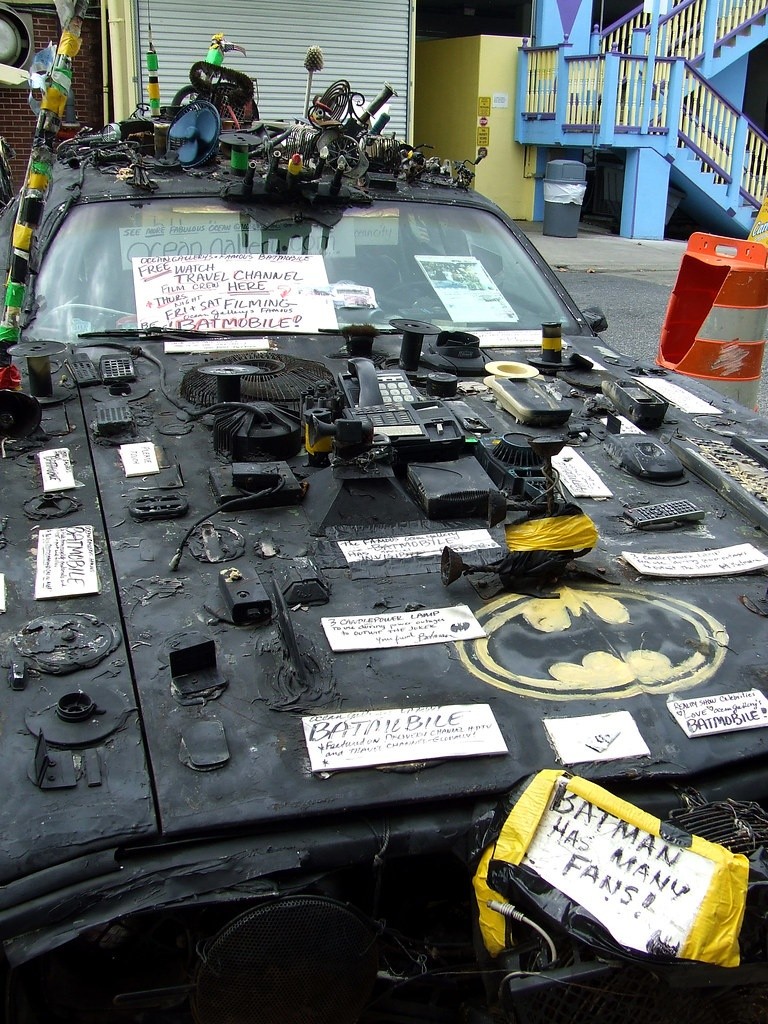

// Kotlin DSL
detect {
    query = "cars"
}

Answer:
[0,35,768,1023]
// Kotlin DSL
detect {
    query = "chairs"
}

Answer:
[342,242,411,289]
[92,250,139,320]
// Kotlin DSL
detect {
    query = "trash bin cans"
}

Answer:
[650,229,768,413]
[541,159,588,238]
